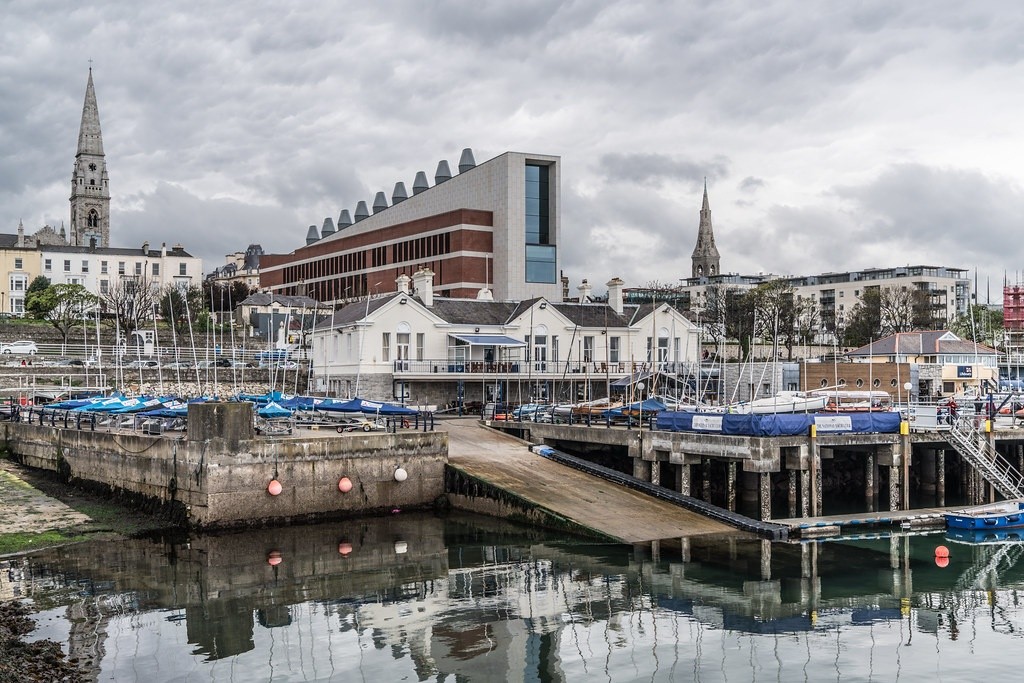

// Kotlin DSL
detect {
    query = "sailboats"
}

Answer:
[0,281,1023,439]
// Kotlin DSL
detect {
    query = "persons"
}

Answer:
[974,392,983,415]
[21,359,33,365]
[937,395,957,425]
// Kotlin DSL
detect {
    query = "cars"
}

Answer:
[257,347,292,362]
[58,358,85,367]
[130,359,159,369]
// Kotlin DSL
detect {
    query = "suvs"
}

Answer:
[1,340,39,357]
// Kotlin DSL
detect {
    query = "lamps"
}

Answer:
[399,299,407,304]
[602,331,606,334]
[475,328,479,332]
[540,303,546,309]
[662,307,671,313]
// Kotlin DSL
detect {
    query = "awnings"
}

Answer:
[448,333,528,347]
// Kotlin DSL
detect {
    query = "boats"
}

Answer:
[941,495,1024,533]
[942,525,1024,546]
